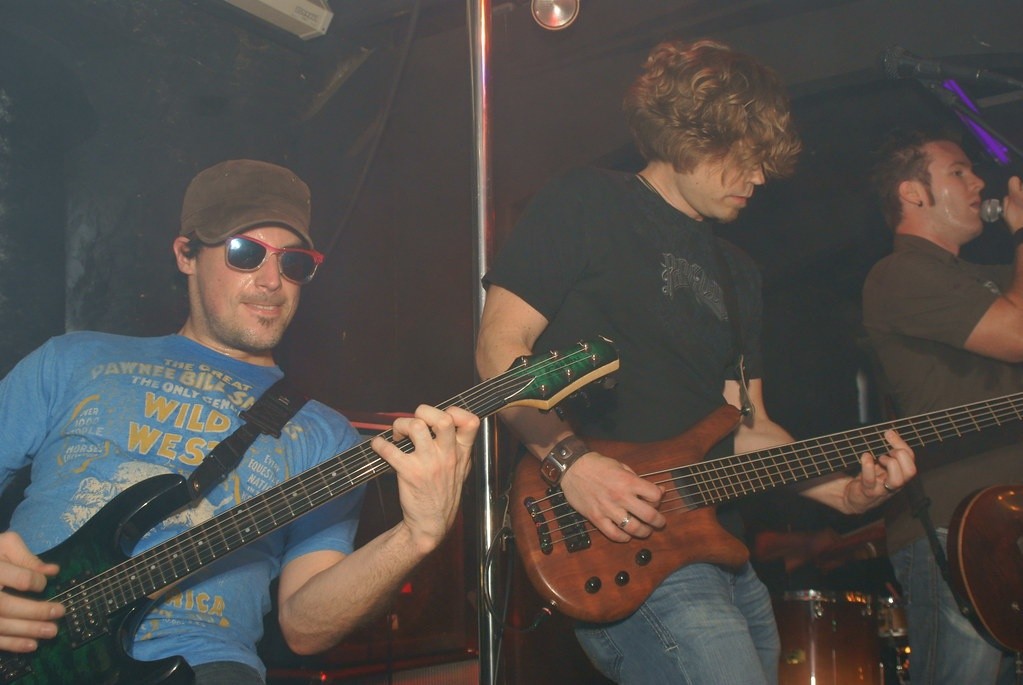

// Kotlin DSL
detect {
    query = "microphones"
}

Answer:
[875,45,1001,88]
[979,199,1005,224]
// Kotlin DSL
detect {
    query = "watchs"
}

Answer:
[538,433,594,489]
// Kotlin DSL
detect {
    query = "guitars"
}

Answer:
[509,389,1023,625]
[946,481,1023,655]
[0,333,622,684]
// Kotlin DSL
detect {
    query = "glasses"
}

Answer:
[191,229,325,284]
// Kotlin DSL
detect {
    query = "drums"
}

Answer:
[762,586,892,683]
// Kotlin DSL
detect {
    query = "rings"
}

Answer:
[618,511,632,528]
[883,476,900,494]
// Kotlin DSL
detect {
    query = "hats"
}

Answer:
[177,158,316,253]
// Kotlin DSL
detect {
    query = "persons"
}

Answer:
[471,34,920,684]
[0,156,481,684]
[861,129,1022,683]
